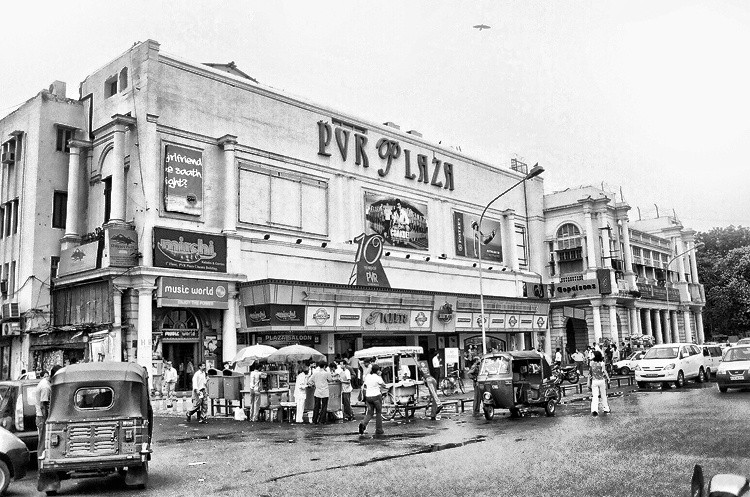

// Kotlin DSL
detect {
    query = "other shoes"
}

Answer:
[295,422,306,424]
[313,421,318,424]
[250,418,258,422]
[343,416,353,422]
[604,410,610,415]
[593,411,598,416]
[199,419,209,424]
[377,431,384,434]
[186,411,191,422]
[359,423,364,434]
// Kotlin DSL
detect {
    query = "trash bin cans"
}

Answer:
[223,369,244,400]
[207,368,224,399]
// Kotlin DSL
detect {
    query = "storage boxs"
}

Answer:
[391,385,416,397]
[306,410,319,424]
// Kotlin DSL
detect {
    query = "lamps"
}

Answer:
[406,254,410,258]
[296,239,302,244]
[322,243,327,247]
[386,252,390,256]
[473,263,476,267]
[426,257,430,261]
[264,234,270,240]
[489,267,492,270]
[502,268,506,271]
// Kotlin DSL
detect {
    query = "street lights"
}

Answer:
[665,242,706,343]
[477,166,546,353]
[696,337,750,382]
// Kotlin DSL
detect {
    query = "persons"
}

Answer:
[615,271,623,283]
[657,279,666,287]
[186,362,209,423]
[432,351,443,390]
[633,271,638,282]
[722,343,732,361]
[464,348,503,367]
[185,357,195,391]
[470,220,496,257]
[359,364,384,434]
[70,356,80,364]
[737,337,742,345]
[293,347,389,426]
[692,337,696,343]
[528,348,543,365]
[369,199,429,247]
[18,370,26,380]
[467,355,483,416]
[222,362,232,371]
[36,365,62,479]
[555,348,562,366]
[571,348,584,376]
[250,364,266,422]
[584,342,646,366]
[163,361,186,408]
[588,351,610,415]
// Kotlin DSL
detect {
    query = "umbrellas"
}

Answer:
[232,343,278,364]
[267,342,327,372]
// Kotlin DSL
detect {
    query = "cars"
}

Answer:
[715,343,750,394]
[611,352,645,373]
[0,378,40,497]
[637,338,708,391]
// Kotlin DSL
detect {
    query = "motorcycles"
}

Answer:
[477,352,565,424]
[40,363,155,496]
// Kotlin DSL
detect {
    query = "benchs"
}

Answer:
[527,374,541,390]
[278,402,298,421]
[245,405,280,424]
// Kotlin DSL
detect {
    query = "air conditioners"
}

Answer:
[2,321,20,335]
